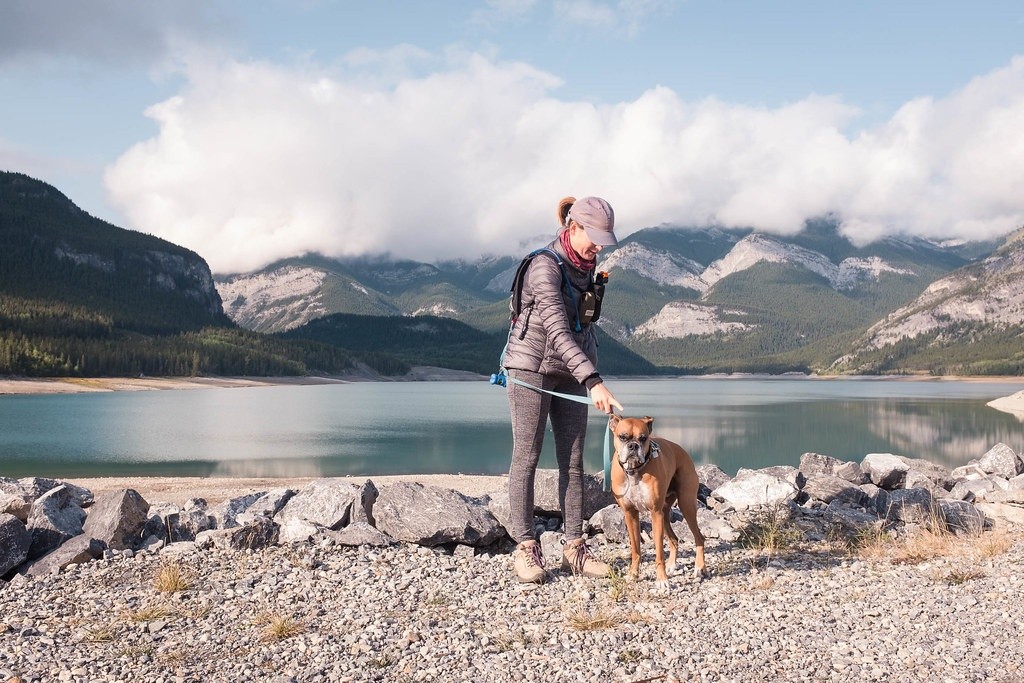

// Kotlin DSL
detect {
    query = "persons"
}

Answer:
[499,197,624,584]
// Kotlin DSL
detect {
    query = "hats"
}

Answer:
[570,196,618,246]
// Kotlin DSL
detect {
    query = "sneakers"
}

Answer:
[514,540,547,582]
[561,537,609,578]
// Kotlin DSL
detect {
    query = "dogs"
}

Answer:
[609,412,708,590]
